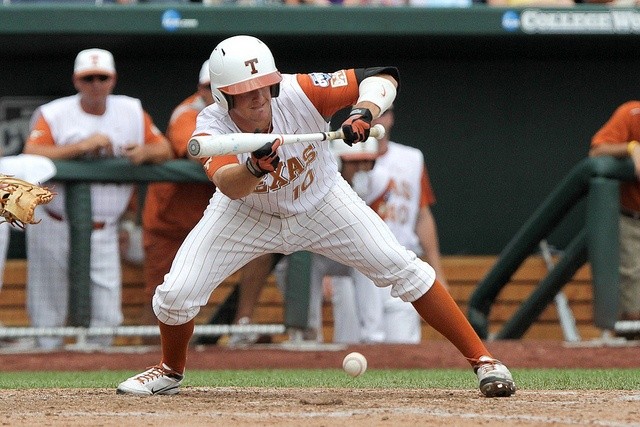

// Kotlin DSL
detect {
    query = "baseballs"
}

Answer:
[343,352,368,377]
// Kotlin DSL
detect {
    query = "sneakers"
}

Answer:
[115,361,186,397]
[472,364,517,398]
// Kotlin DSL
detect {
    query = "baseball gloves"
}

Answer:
[0,173,58,229]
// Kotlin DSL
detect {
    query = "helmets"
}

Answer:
[208,35,283,116]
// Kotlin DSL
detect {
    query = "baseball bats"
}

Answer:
[187,123,385,158]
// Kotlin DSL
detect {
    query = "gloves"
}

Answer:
[246,139,282,179]
[340,108,373,148]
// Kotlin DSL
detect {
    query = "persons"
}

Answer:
[114,33,517,397]
[24,48,173,347]
[330,97,448,343]
[118,186,144,267]
[142,59,278,344]
[590,100,640,336]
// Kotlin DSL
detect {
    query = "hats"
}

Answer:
[200,60,212,85]
[74,49,116,77]
